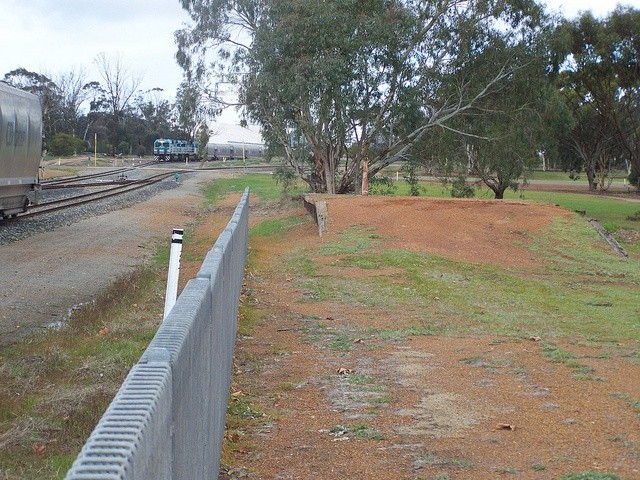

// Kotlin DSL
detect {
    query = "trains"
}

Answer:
[152,137,266,162]
[0,82,41,218]
